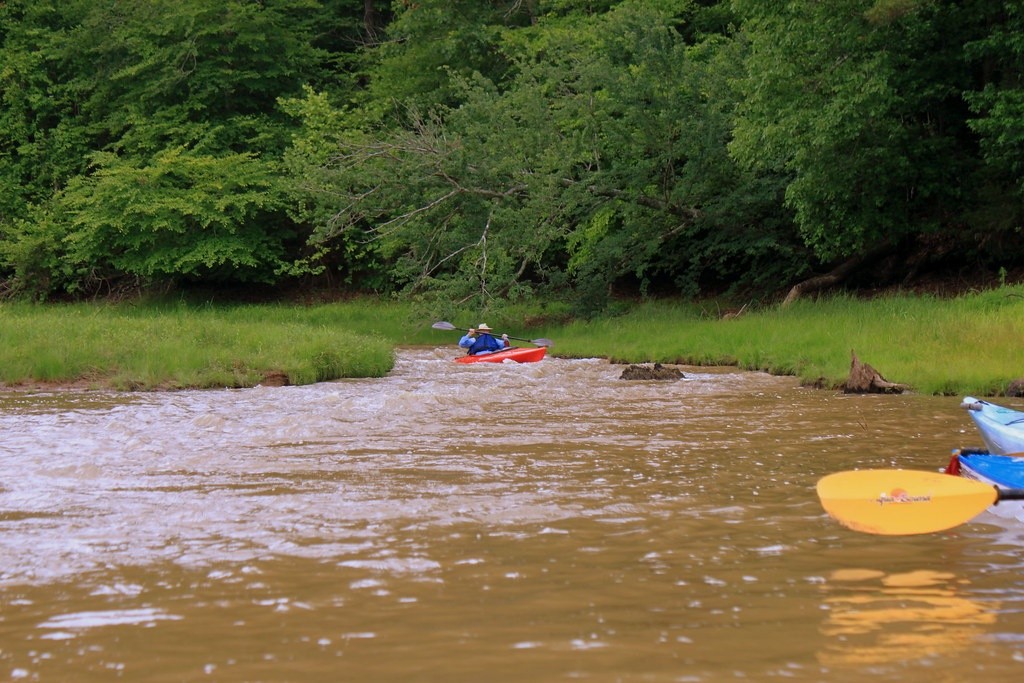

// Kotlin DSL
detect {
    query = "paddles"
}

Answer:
[813,467,1024,536]
[431,321,554,346]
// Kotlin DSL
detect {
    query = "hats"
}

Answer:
[476,323,493,330]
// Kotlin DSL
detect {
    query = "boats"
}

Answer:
[451,345,547,364]
[951,446,1024,500]
[963,396,1024,455]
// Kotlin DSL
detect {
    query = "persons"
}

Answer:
[459,324,510,355]
